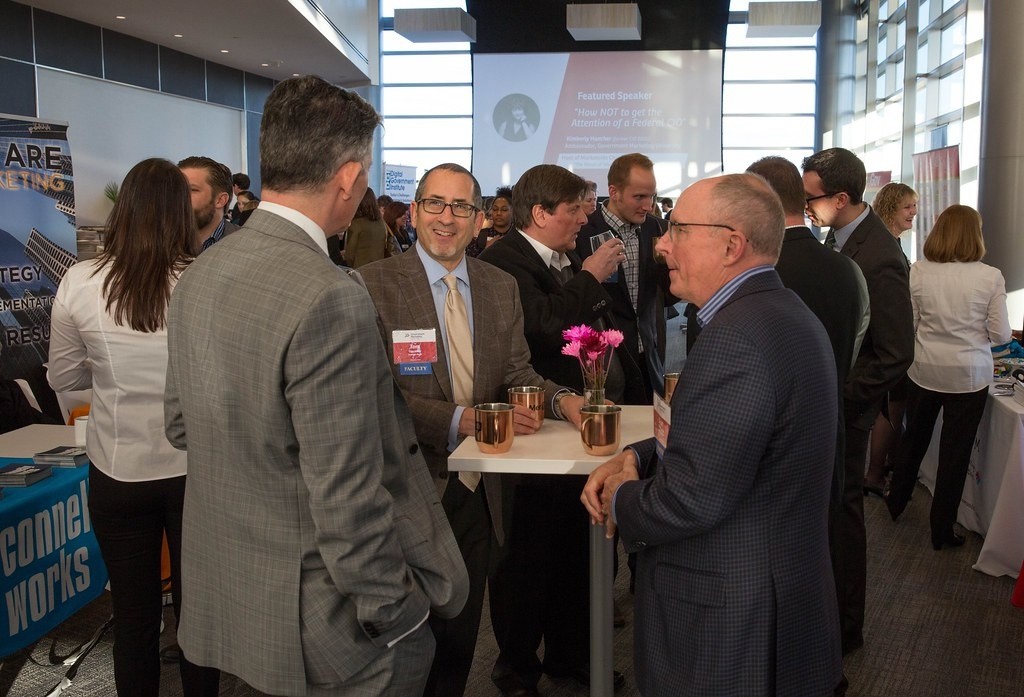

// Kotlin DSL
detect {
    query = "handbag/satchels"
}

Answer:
[382,217,404,261]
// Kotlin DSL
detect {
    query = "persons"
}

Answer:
[862,183,917,500]
[883,205,1013,551]
[802,147,914,661]
[46,158,220,696]
[329,189,410,270]
[498,104,535,141]
[164,76,469,696]
[176,156,261,252]
[580,174,851,697]
[471,152,674,697]
[747,156,871,518]
[341,164,616,696]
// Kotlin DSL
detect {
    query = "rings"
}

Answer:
[602,510,607,515]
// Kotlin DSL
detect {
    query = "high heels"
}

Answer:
[885,490,900,523]
[933,531,967,551]
[864,479,912,502]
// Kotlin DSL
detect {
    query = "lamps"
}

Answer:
[747,2,822,38]
[566,0,642,41]
[394,8,476,43]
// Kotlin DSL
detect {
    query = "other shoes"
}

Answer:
[614,611,627,628]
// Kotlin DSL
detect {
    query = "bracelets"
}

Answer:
[521,120,524,123]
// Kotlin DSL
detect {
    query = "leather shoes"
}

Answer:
[542,653,625,692]
[503,680,541,697]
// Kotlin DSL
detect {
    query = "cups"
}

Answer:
[580,405,622,456]
[663,373,681,403]
[74,416,88,446]
[473,403,515,454]
[590,230,622,266]
[508,386,545,434]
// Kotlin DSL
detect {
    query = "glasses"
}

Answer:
[804,188,849,209]
[416,196,482,217]
[667,220,750,246]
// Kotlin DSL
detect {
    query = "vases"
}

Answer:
[583,386,606,405]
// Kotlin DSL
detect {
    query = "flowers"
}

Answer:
[561,324,624,404]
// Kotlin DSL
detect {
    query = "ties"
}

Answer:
[826,233,837,248]
[441,274,482,492]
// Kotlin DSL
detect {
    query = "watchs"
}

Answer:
[555,392,574,421]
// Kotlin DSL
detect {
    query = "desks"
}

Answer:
[902,357,1024,579]
[447,405,655,697]
[0,424,110,660]
